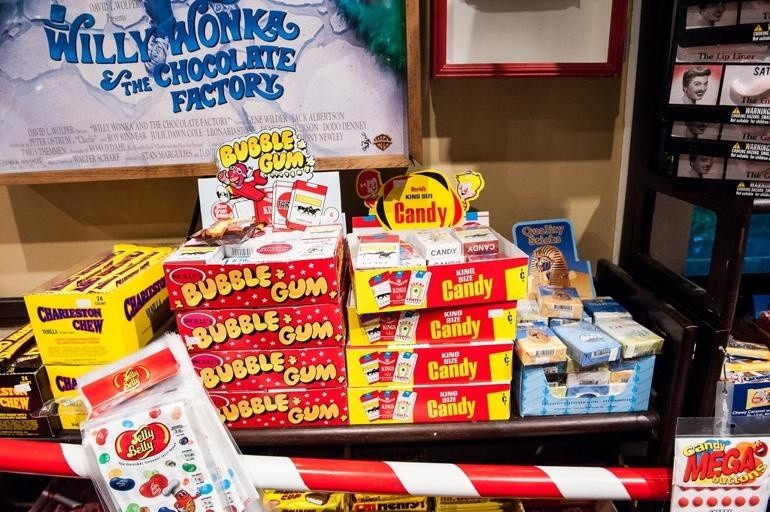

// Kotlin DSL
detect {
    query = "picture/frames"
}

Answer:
[0,0,422,191]
[430,1,628,77]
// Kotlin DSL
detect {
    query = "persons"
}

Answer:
[683,122,708,137]
[688,155,713,178]
[681,66,711,104]
[697,0,725,26]
